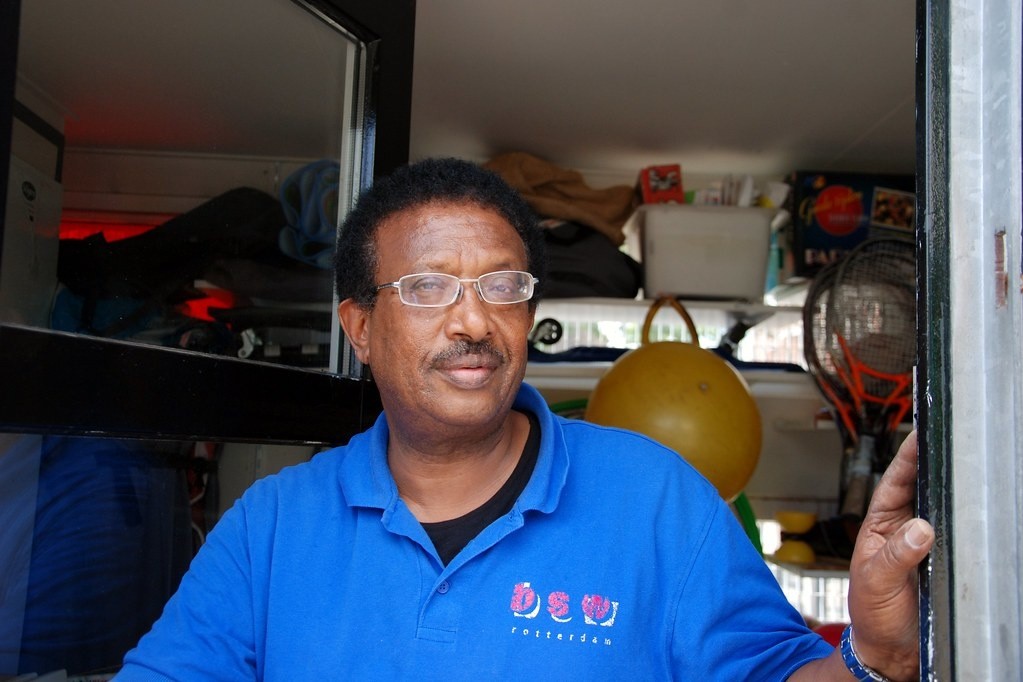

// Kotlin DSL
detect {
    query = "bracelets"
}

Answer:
[839,623,889,682]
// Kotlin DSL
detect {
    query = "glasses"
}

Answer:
[374,271,539,309]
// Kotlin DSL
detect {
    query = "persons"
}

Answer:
[106,156,935,682]
[0,257,193,682]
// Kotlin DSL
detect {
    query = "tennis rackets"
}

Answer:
[803,235,918,516]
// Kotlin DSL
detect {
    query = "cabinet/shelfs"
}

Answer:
[212,262,917,656]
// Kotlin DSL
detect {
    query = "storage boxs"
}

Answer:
[638,204,775,301]
